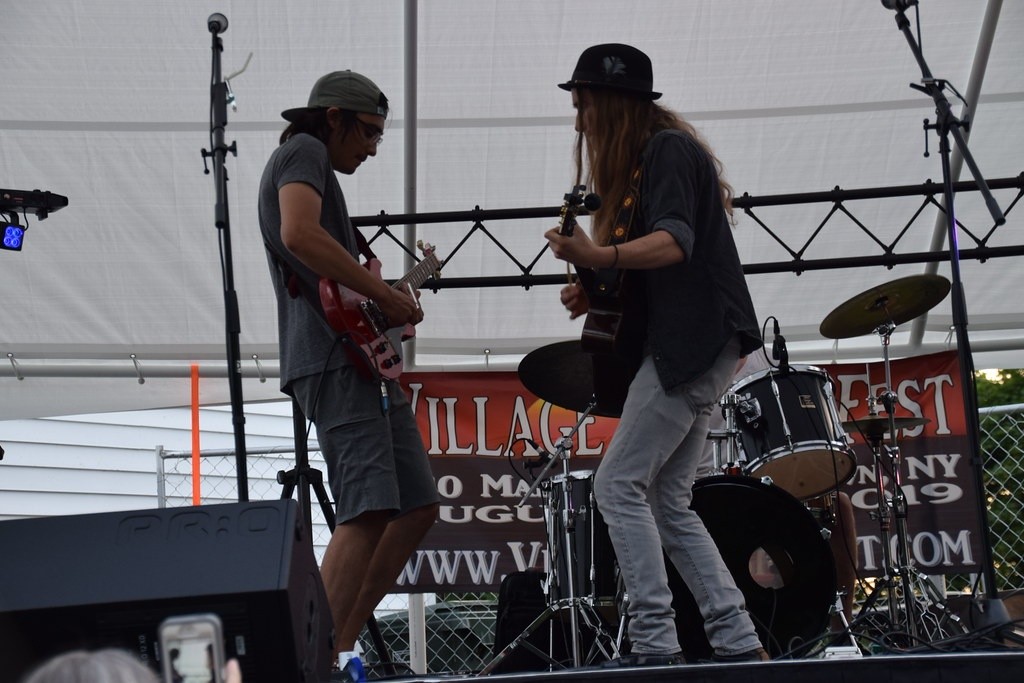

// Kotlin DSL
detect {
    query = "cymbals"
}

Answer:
[518,340,622,418]
[819,275,951,339]
[842,415,932,433]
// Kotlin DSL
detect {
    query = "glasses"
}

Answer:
[355,116,383,145]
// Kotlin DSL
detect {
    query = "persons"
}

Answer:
[545,43,772,669]
[257,70,442,672]
[694,356,873,657]
[25,650,160,683]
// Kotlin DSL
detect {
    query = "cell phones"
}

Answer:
[158,614,226,683]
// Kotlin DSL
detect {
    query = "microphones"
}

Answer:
[881,0,918,10]
[208,13,229,34]
[526,436,558,469]
[772,322,784,360]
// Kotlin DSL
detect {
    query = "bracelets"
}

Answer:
[604,244,619,271]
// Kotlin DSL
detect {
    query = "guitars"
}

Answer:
[559,185,646,412]
[319,240,442,383]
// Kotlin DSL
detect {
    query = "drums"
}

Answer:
[617,475,838,663]
[546,469,618,627]
[720,365,858,503]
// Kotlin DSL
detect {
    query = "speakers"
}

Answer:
[0,498,337,683]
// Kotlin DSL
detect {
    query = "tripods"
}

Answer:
[474,394,622,677]
[832,9,1024,656]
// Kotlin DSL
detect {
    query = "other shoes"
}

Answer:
[698,648,770,664]
[600,651,686,668]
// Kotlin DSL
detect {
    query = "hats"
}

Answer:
[557,44,662,100]
[282,70,388,122]
